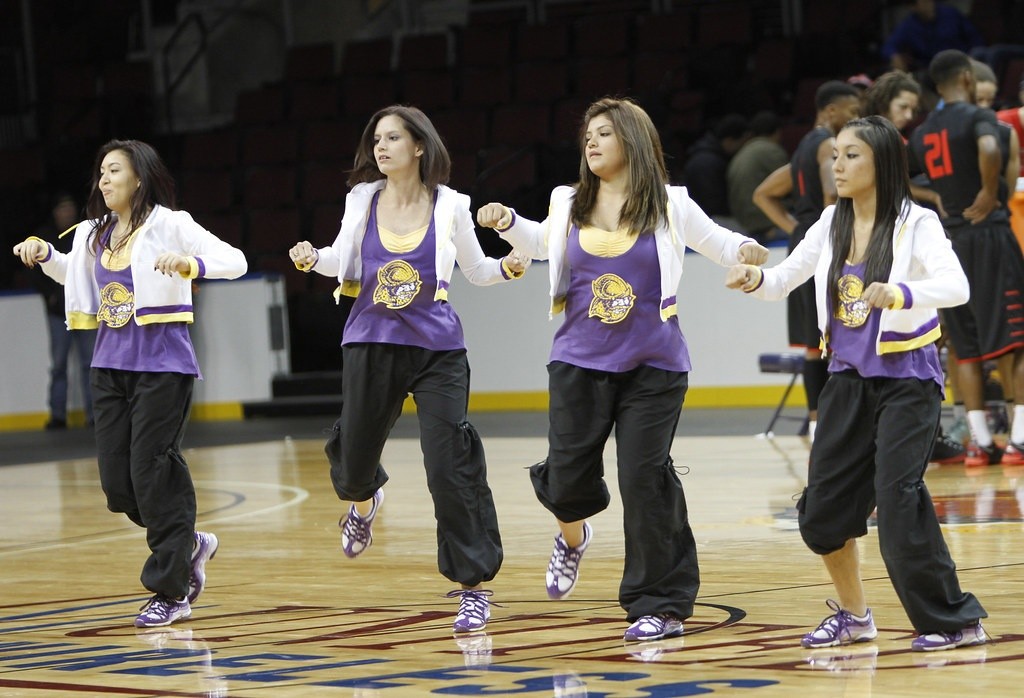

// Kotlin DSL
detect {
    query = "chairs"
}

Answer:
[759,354,805,435]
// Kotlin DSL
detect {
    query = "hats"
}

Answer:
[847,73,874,88]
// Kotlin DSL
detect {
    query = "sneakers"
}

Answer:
[443,589,510,633]
[912,651,986,670]
[135,593,191,628]
[804,647,878,678]
[624,641,684,662]
[964,439,1004,466]
[453,631,493,676]
[339,487,385,558]
[623,612,684,642]
[911,618,986,652]
[187,531,220,604]
[800,599,877,648]
[928,435,967,464]
[545,521,593,600]
[1001,440,1024,465]
[554,668,587,698]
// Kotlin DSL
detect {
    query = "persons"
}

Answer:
[684,0,1024,468]
[287,104,531,637]
[475,98,770,642]
[11,137,251,629]
[723,115,992,653]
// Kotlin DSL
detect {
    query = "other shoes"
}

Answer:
[46,418,67,429]
